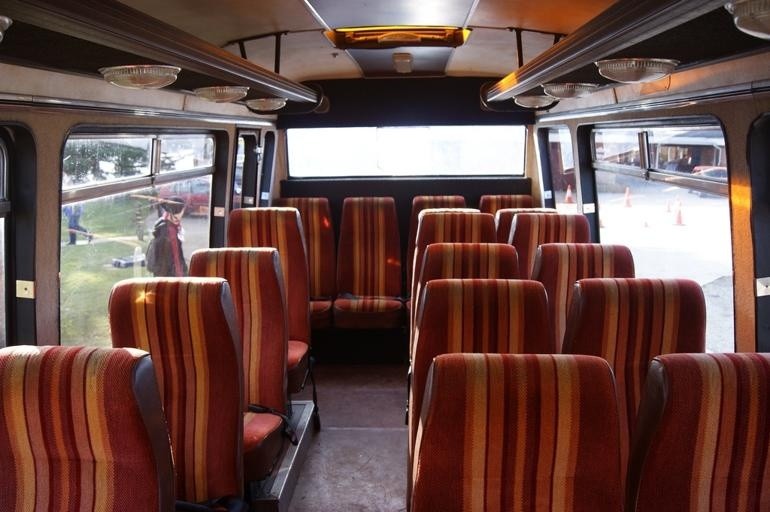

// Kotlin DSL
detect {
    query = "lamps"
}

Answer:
[0,16,289,111]
[512,0,770,108]
[392,53,414,73]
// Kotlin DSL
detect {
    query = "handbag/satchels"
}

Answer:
[148,237,172,274]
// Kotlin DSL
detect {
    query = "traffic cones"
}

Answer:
[562,184,576,204]
[622,186,632,208]
[676,202,685,226]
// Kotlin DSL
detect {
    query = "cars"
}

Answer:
[154,175,242,218]
[687,166,729,197]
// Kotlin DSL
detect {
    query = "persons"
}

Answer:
[679,154,689,173]
[145,195,188,277]
[60,200,93,245]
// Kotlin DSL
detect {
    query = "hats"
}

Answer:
[160,197,185,214]
